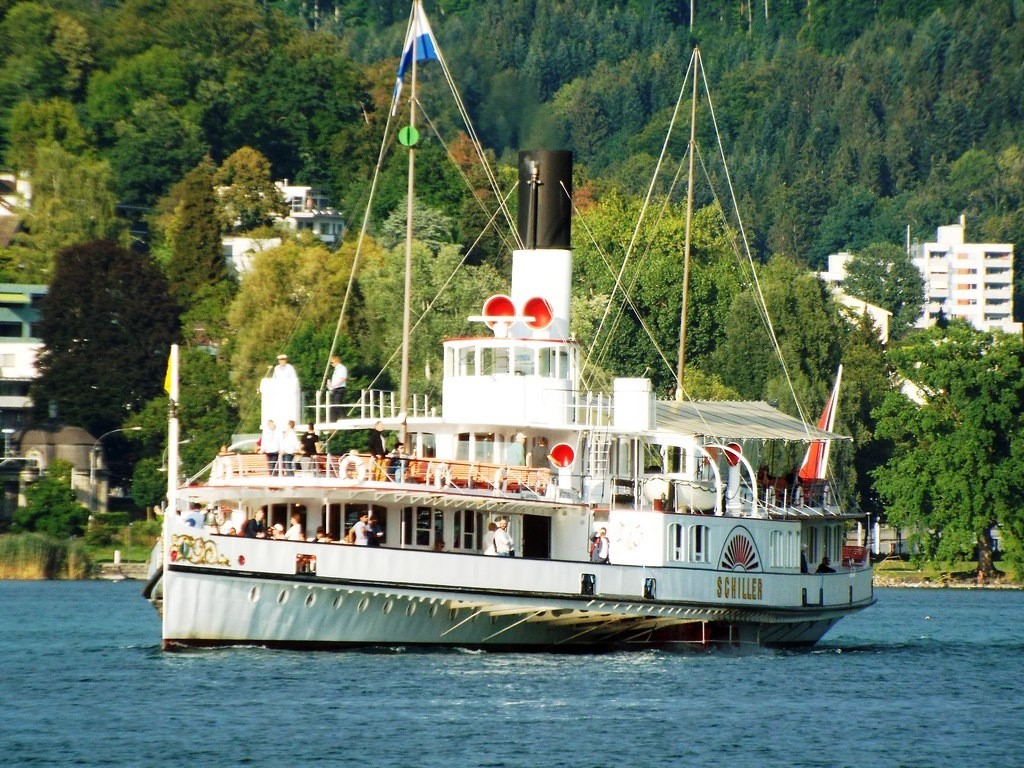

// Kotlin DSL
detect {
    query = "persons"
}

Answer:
[507,433,526,466]
[259,419,322,477]
[757,466,773,479]
[801,544,808,573]
[494,519,515,556]
[432,540,444,550]
[272,355,296,377]
[589,527,610,563]
[526,437,551,470]
[329,356,347,421]
[368,421,410,482]
[784,464,803,497]
[815,557,836,573]
[483,522,497,554]
[177,503,379,546]
[218,444,236,456]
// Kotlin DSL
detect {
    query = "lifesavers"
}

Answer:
[218,457,232,486]
[338,455,365,487]
[434,463,452,491]
[494,467,508,495]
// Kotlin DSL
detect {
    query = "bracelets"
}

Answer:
[349,541,352,543]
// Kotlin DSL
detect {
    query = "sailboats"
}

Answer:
[142,0,877,654]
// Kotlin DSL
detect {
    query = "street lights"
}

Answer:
[90,426,143,510]
[162,439,190,470]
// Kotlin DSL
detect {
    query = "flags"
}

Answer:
[798,388,838,478]
[392,0,439,116]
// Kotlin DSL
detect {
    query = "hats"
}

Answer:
[277,355,286,360]
